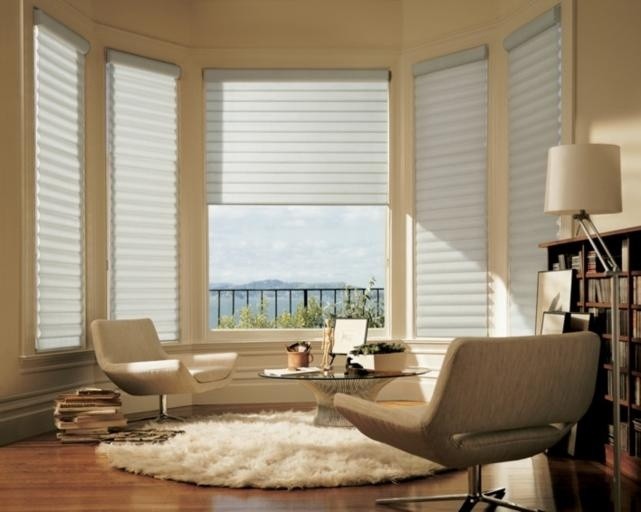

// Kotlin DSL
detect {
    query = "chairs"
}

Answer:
[91,317,240,430]
[334,330,603,511]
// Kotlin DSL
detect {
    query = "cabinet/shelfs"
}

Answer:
[539,226,641,486]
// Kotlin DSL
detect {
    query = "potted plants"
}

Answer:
[346,340,413,376]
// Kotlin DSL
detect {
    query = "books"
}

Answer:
[263,367,320,377]
[552,250,641,456]
[53,387,185,445]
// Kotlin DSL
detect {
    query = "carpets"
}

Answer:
[94,408,452,487]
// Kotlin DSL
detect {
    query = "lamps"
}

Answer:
[543,143,624,511]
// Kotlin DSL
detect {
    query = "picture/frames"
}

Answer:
[533,270,594,460]
